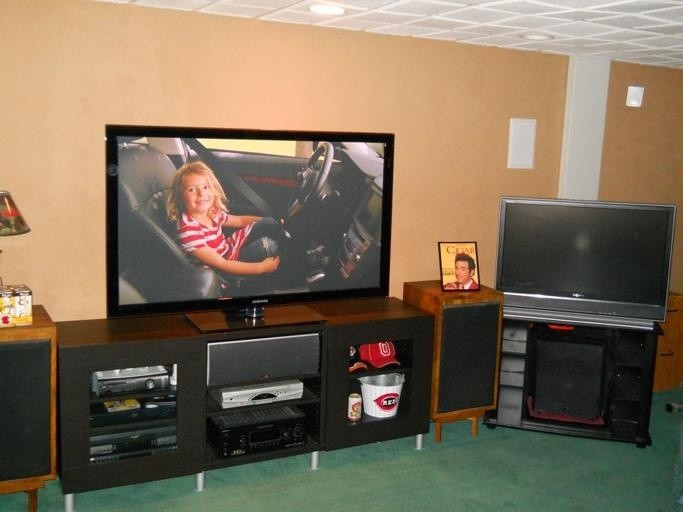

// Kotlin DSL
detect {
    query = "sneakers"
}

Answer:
[305,242,326,258]
[304,268,327,285]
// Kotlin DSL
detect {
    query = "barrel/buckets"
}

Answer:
[356,373,407,419]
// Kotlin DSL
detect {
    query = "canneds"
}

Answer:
[348,393,361,422]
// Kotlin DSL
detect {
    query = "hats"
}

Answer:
[347,344,369,374]
[358,340,402,369]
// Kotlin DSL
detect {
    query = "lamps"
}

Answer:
[0,190,31,287]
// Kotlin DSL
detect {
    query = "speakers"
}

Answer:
[534,330,607,420]
[206,332,321,389]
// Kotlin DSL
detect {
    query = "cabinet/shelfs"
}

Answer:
[483,304,662,450]
[53,295,435,508]
[651,291,681,393]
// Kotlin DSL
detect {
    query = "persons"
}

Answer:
[167,162,327,284]
[445,252,479,289]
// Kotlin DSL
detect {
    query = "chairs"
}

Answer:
[116,135,228,301]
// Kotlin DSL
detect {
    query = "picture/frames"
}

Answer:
[437,240,483,292]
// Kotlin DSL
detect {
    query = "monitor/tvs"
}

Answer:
[496,196,678,332]
[104,124,395,317]
